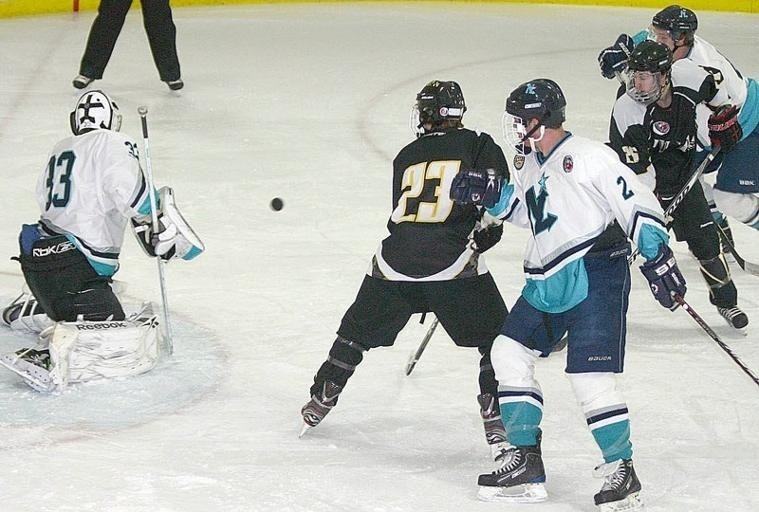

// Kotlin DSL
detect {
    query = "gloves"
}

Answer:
[595,34,634,80]
[640,249,687,311]
[471,223,504,254]
[450,170,502,210]
[134,209,179,258]
[704,105,743,150]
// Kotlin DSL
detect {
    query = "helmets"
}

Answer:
[626,41,673,76]
[648,5,699,48]
[415,79,465,131]
[70,91,122,137]
[504,79,568,131]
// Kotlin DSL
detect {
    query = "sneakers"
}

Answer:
[716,304,750,329]
[16,347,54,370]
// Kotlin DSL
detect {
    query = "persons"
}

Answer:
[72,0,184,91]
[1,88,205,388]
[609,39,749,330]
[448,76,688,506]
[299,78,512,446]
[597,3,759,255]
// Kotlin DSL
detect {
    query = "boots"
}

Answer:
[166,78,183,91]
[592,458,642,504]
[475,392,508,444]
[716,219,734,251]
[302,380,342,428]
[474,430,546,485]
[72,73,95,89]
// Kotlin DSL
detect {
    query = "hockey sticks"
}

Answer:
[714,219,758,276]
[138,107,175,358]
[406,314,439,375]
